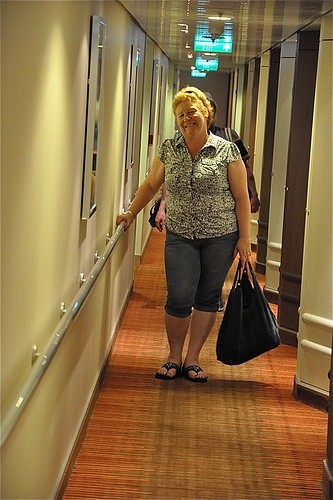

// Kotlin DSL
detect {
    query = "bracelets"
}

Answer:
[127,209,135,219]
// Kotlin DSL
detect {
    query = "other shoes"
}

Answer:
[217,297,225,311]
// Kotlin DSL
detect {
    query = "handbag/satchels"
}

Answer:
[148,198,168,228]
[216,259,281,367]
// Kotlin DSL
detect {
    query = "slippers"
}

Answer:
[155,362,181,380]
[181,363,208,383]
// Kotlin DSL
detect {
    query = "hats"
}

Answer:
[202,90,218,115]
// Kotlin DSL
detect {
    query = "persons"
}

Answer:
[115,87,280,382]
